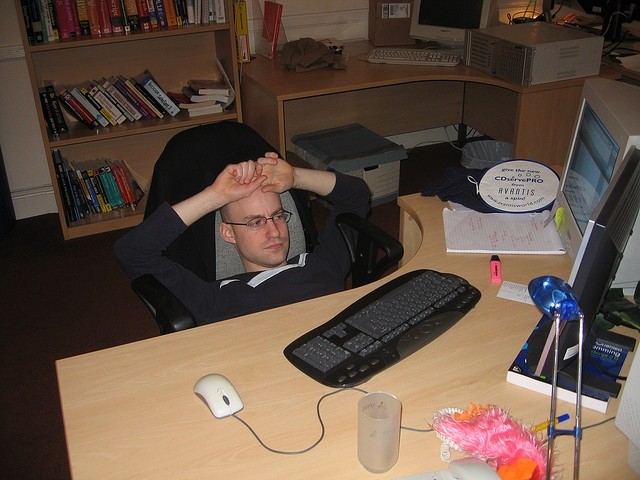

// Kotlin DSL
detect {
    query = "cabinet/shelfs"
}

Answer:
[14,1,244,243]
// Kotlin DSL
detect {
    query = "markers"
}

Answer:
[490,255,501,282]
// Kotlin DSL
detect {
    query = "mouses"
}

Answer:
[192,372,243,418]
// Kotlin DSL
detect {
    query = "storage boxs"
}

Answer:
[290,120,409,210]
[367,0,416,48]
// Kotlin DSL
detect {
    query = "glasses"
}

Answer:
[225,209,292,230]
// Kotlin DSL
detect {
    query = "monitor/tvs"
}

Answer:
[532,145,640,398]
[546,74,640,298]
[408,0,493,53]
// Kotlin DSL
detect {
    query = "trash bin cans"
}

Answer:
[461,141,513,168]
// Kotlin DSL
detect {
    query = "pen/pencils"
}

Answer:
[531,414,569,432]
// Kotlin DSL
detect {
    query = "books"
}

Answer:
[234,1,251,64]
[52,148,145,223]
[39,69,180,137]
[168,79,230,118]
[256,0,283,61]
[506,314,629,414]
[441,204,568,257]
[20,0,226,47]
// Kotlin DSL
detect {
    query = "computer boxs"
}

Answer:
[463,19,606,87]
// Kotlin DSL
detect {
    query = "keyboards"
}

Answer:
[283,267,483,389]
[367,46,461,68]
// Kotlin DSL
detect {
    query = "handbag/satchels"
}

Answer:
[281,37,349,75]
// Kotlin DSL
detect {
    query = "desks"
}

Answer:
[50,192,639,479]
[238,37,601,170]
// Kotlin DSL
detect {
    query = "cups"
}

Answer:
[332,52,353,71]
[357,392,402,473]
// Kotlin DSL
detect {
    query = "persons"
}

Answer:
[112,152,371,327]
